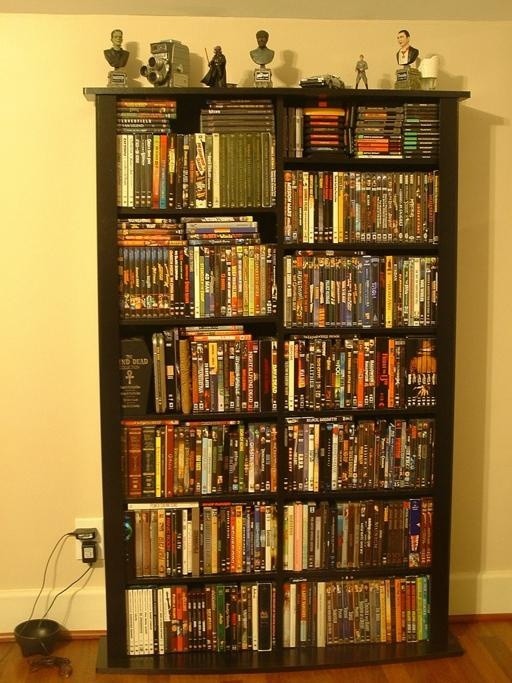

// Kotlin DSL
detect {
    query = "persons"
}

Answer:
[103,28,131,68]
[393,29,420,68]
[248,30,275,66]
[353,54,370,90]
[198,45,228,89]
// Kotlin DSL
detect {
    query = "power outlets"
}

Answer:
[75,518,104,561]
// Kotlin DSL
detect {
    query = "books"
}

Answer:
[112,94,438,657]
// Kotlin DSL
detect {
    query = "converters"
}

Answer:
[71,528,97,540]
[81,546,96,564]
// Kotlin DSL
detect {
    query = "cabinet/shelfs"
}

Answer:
[83,87,471,673]
